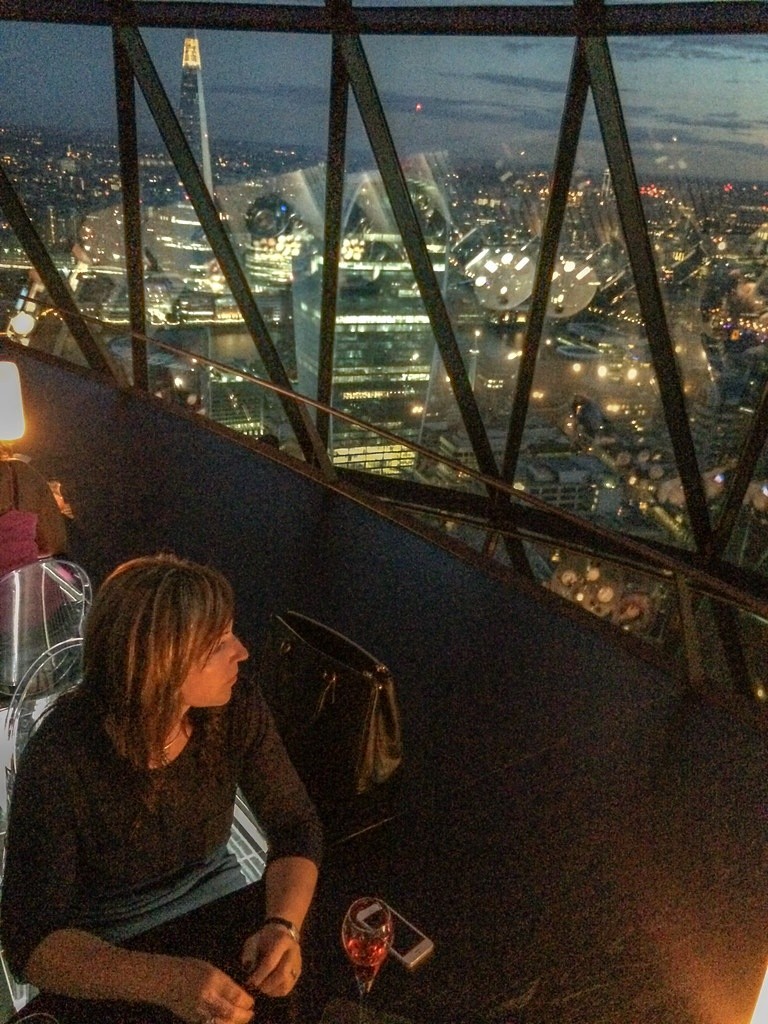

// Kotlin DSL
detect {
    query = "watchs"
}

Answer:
[264,916,301,943]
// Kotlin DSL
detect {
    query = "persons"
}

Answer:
[0,458,87,689]
[0,548,325,1024]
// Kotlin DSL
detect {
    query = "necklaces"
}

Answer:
[164,727,182,749]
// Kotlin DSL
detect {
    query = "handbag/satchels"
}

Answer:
[261,608,403,795]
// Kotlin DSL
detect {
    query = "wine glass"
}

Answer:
[341,897,395,1024]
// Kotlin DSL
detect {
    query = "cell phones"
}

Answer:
[355,900,435,967]
